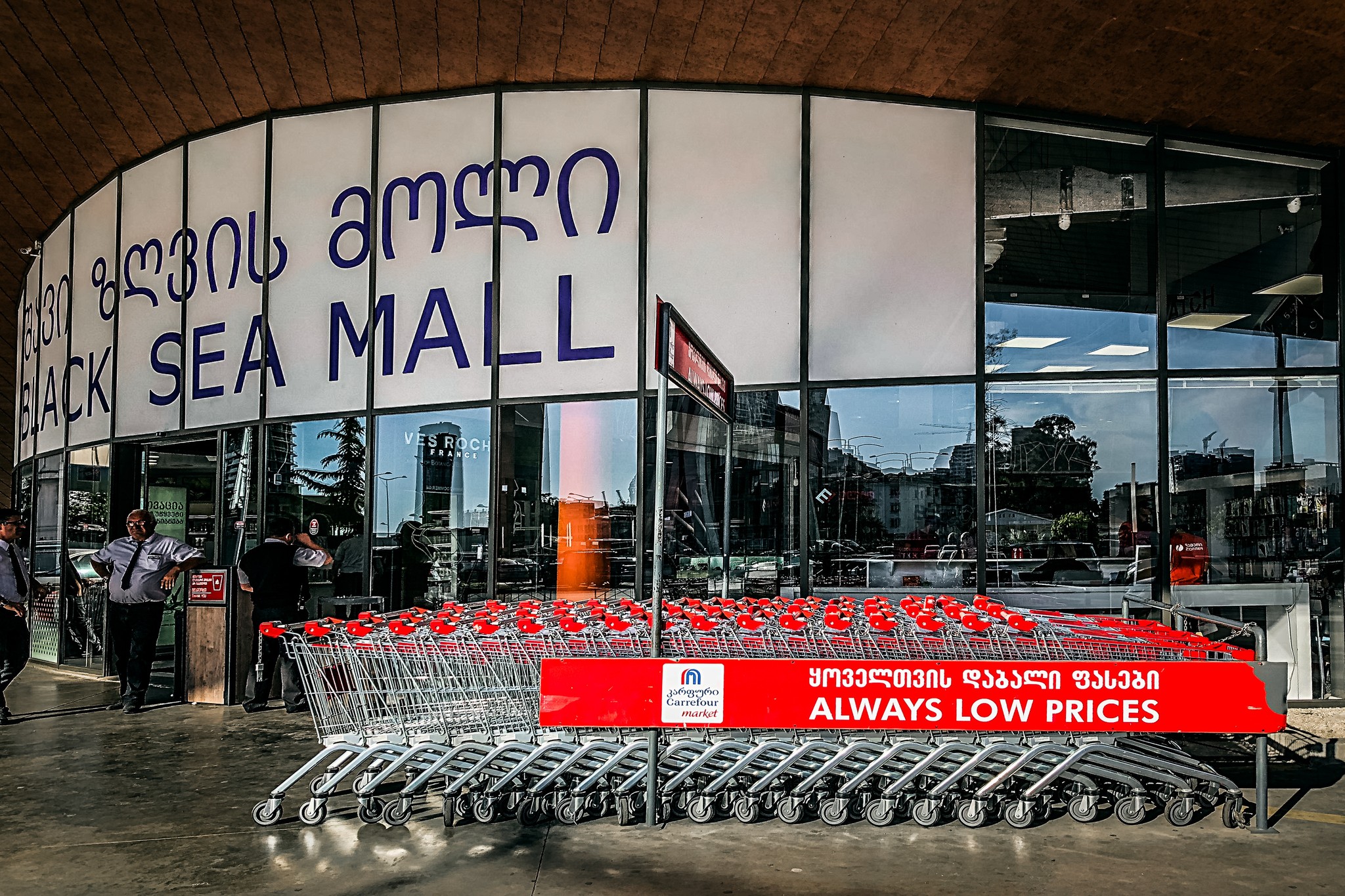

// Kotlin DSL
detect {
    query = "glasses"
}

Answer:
[2,519,26,526]
[126,519,152,526]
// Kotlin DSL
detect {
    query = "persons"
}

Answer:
[236,517,333,713]
[1117,502,1209,632]
[89,509,206,713]
[906,515,989,559]
[0,508,47,726]
[66,556,102,657]
[380,520,437,613]
[332,522,364,619]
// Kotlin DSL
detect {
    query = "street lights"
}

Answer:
[567,492,595,525]
[869,451,949,473]
[378,472,407,538]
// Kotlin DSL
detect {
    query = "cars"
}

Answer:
[759,537,892,562]
[924,543,966,572]
[459,556,541,583]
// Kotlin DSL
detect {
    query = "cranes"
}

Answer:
[1201,430,1218,453]
[914,421,976,444]
[1219,438,1229,448]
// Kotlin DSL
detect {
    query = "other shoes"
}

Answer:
[0,705,11,725]
[123,702,138,713]
[106,702,123,710]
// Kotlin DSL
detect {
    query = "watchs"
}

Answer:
[0,599,9,607]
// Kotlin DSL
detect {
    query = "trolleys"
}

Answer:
[36,575,105,654]
[251,594,1255,830]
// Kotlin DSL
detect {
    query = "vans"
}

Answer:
[1006,540,1104,585]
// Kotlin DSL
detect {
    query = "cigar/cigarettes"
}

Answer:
[15,614,18,616]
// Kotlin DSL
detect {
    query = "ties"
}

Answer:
[8,544,28,598]
[121,543,144,591]
